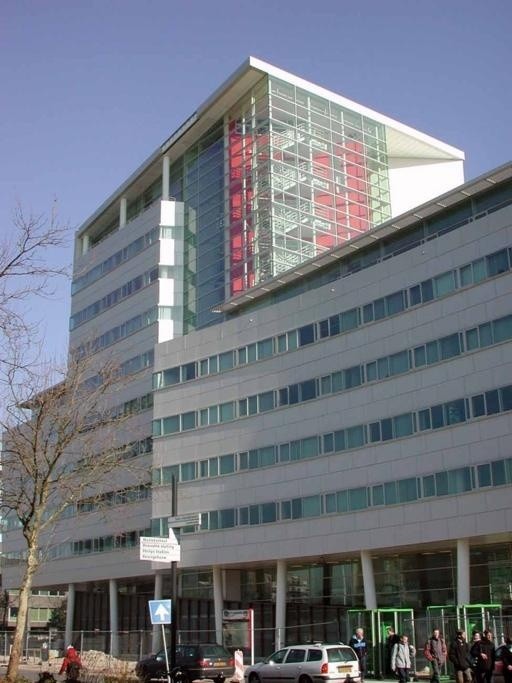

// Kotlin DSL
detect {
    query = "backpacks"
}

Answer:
[67,661,79,680]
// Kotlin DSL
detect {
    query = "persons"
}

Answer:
[348,627,368,683]
[381,627,401,678]
[448,629,472,682]
[467,631,483,682]
[471,628,496,682]
[502,635,511,683]
[391,634,416,683]
[424,629,447,682]
[59,645,83,682]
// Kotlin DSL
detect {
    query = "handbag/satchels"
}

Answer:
[465,654,480,670]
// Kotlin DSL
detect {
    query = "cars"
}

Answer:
[244,642,362,683]
[135,644,235,682]
